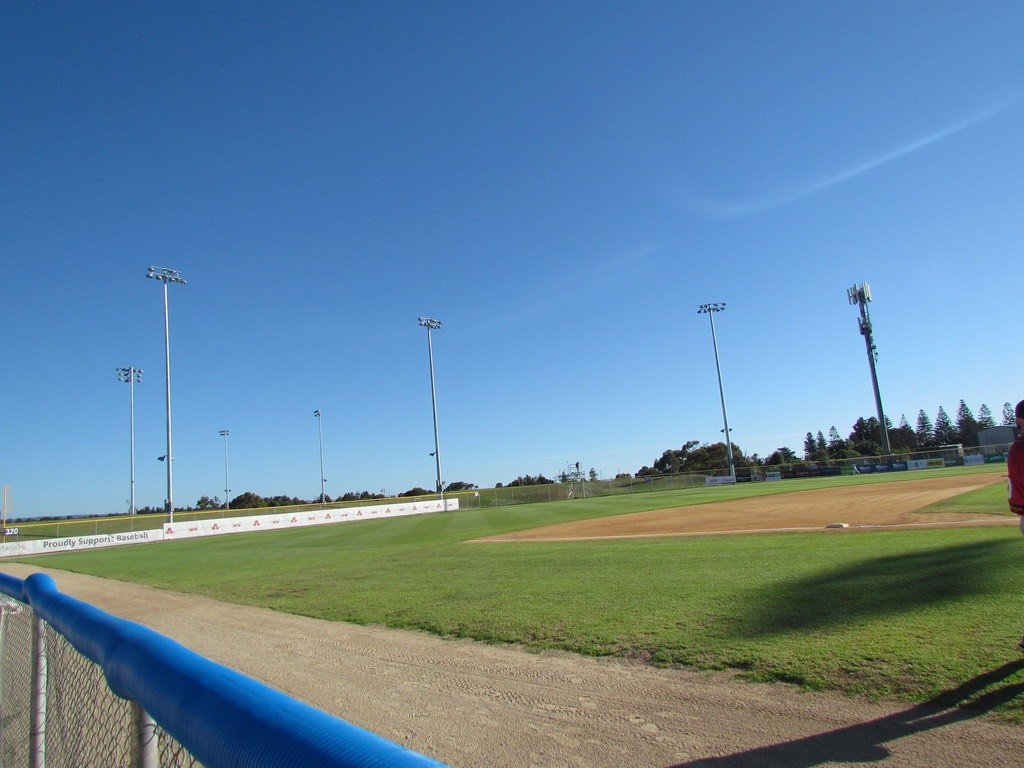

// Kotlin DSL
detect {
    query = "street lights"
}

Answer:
[116,366,144,515]
[146,264,188,523]
[698,302,735,476]
[418,316,444,494]
[314,409,326,503]
[218,429,229,509]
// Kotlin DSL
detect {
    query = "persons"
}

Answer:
[1007,400,1024,654]
